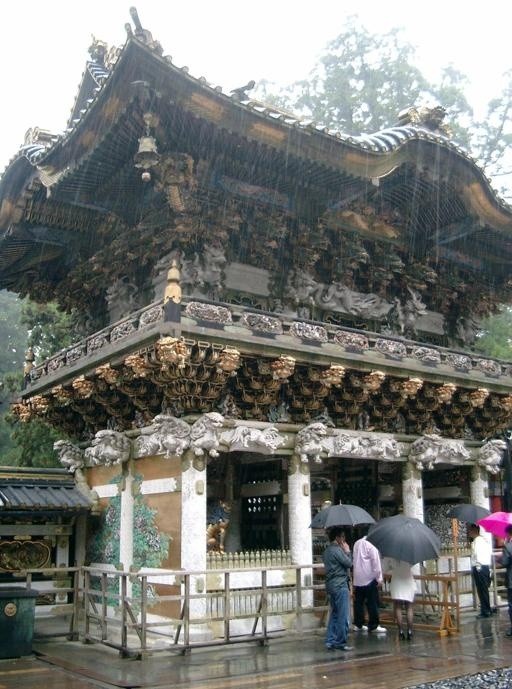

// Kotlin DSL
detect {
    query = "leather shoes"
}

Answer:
[476,610,492,618]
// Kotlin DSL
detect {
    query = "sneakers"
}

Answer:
[326,646,355,651]
[371,625,387,633]
[353,624,368,632]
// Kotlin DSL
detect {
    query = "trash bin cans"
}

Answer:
[0,586,39,659]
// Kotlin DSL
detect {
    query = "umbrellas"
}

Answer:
[444,504,492,525]
[308,499,376,529]
[364,513,442,565]
[474,511,512,539]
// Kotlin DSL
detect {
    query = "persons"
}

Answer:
[465,521,492,617]
[382,556,416,641]
[352,525,388,634]
[322,527,355,650]
[497,525,512,636]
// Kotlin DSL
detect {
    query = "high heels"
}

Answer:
[399,629,414,640]
